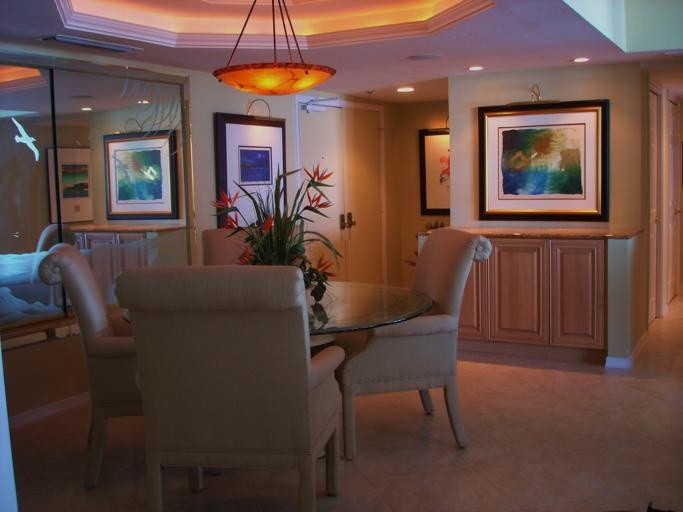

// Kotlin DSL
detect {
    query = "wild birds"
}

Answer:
[10,116,41,164]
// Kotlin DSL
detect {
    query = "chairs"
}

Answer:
[41,243,143,488]
[332,227,494,461]
[116,265,346,511]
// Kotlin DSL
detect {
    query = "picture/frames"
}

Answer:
[45,130,177,224]
[477,99,610,222]
[417,128,450,216]
[212,111,286,229]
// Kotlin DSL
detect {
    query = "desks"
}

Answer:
[303,274,436,333]
[63,224,191,285]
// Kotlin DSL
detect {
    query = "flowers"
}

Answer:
[208,161,343,303]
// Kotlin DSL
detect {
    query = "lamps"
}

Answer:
[211,0,337,96]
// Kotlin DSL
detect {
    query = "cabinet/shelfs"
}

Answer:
[489,239,606,350]
[457,260,487,338]
